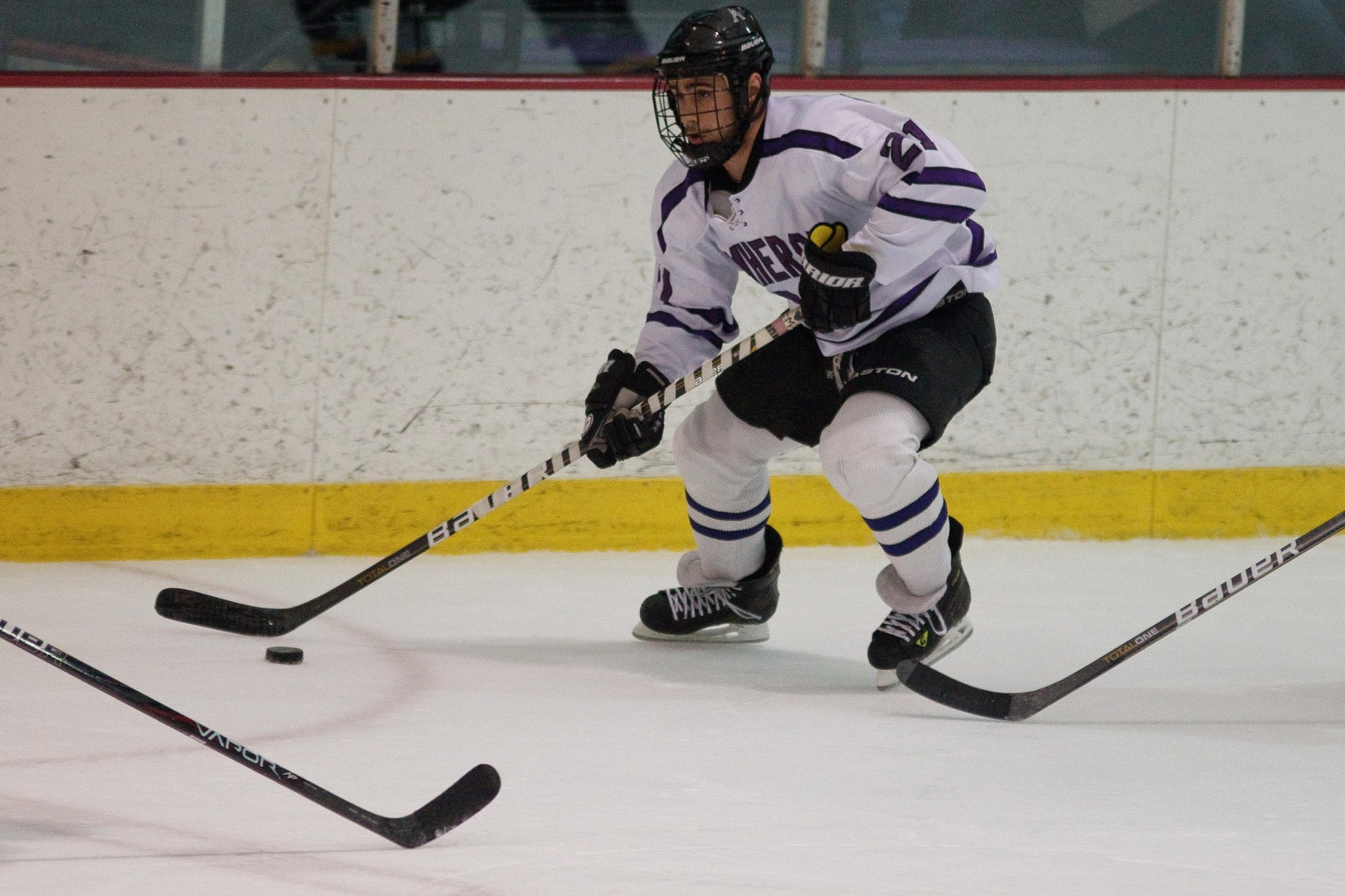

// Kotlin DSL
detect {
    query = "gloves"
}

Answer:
[799,222,877,334]
[580,348,673,470]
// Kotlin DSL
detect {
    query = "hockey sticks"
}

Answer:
[896,504,1345,720]
[0,618,505,853]
[153,303,808,637]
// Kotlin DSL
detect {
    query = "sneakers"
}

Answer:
[631,523,783,644]
[866,516,976,691]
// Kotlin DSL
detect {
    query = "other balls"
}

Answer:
[263,645,305,666]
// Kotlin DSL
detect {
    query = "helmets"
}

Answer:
[652,4,775,161]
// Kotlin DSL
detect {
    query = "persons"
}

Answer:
[580,4,998,688]
[289,0,664,75]
